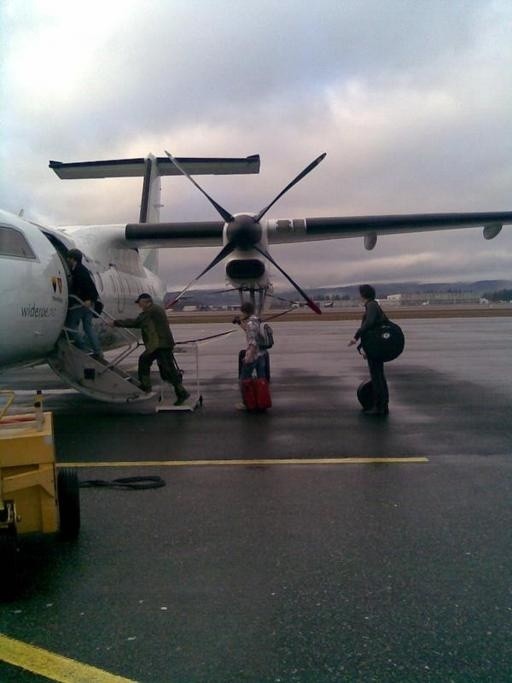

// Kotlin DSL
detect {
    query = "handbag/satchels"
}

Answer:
[173,352,184,384]
[94,302,103,318]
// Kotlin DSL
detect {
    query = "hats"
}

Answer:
[135,293,150,303]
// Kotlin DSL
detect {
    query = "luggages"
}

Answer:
[239,361,272,412]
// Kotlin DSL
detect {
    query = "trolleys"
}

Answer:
[155,340,203,413]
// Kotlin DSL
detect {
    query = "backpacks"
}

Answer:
[360,301,405,361]
[251,318,274,349]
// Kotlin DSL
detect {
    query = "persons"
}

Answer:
[104,292,191,406]
[345,281,392,417]
[65,246,103,362]
[235,300,270,379]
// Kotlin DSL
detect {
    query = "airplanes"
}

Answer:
[0,153,511,404]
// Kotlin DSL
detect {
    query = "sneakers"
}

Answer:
[175,390,190,405]
[71,342,84,349]
[90,351,103,359]
[137,385,153,392]
